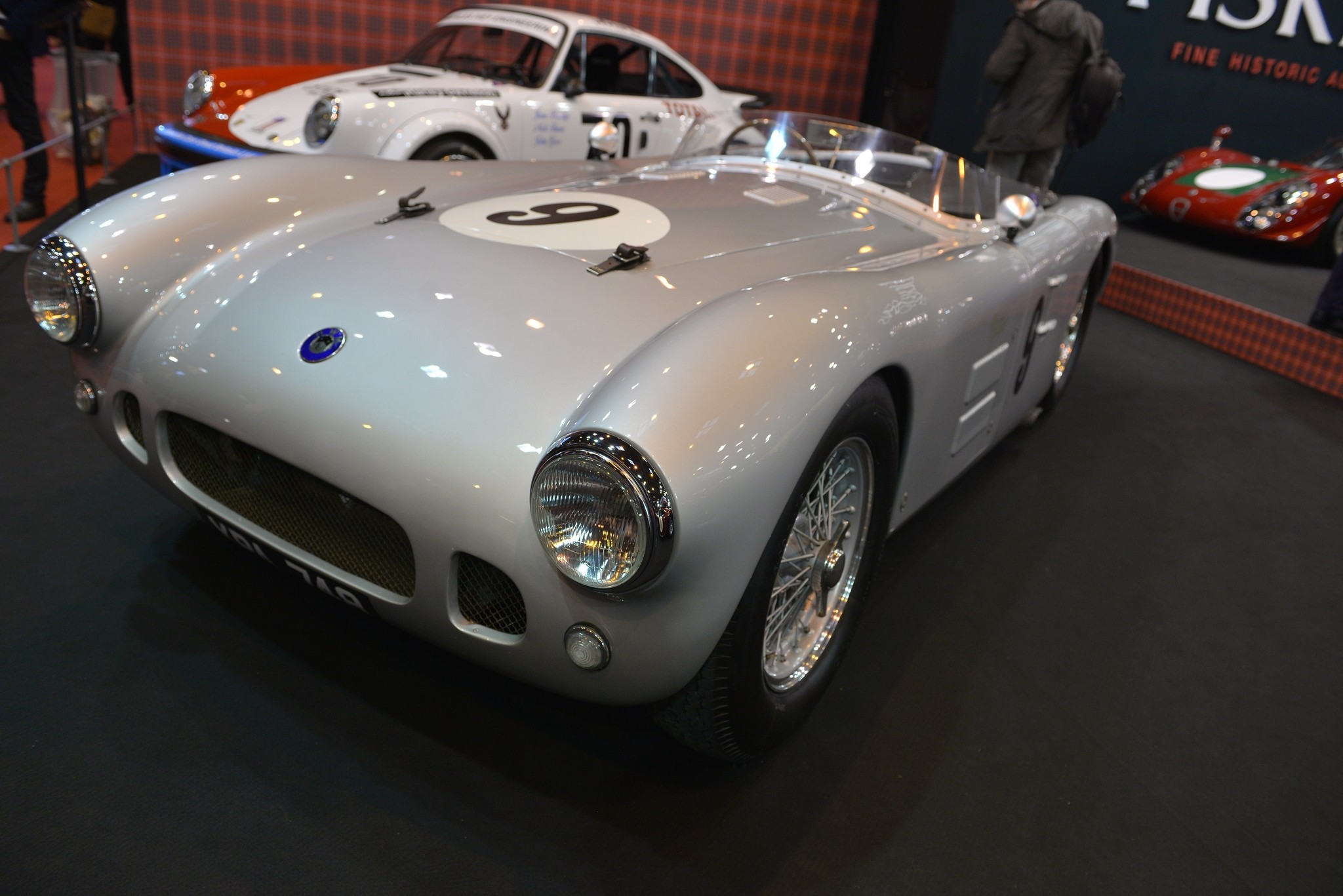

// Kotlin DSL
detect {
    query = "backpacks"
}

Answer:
[1065,11,1126,152]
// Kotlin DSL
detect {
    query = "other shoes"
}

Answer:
[4,200,46,222]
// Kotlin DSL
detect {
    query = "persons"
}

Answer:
[0,1,51,223]
[974,0,1106,190]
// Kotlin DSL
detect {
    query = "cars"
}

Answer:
[139,7,759,191]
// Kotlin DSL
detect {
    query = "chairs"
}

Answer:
[585,42,618,93]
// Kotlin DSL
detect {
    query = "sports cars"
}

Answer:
[1125,124,1343,262]
[16,99,1123,730]
